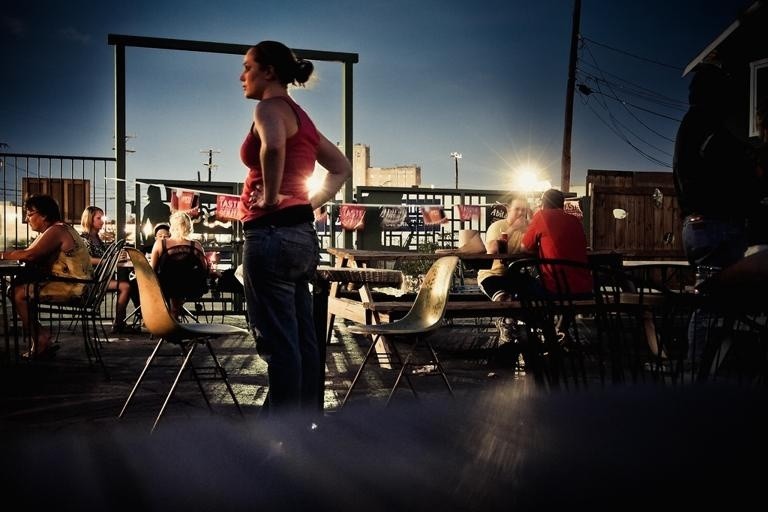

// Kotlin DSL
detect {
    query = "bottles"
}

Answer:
[694,265,712,296]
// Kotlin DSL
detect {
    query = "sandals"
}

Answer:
[22,336,61,359]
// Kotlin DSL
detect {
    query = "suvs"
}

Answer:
[210,244,234,273]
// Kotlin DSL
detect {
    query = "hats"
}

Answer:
[154,223,170,236]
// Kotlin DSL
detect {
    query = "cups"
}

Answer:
[495,239,506,256]
[457,230,475,248]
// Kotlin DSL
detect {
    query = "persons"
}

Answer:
[0,194,95,364]
[238,40,352,420]
[76,205,143,332]
[669,66,756,378]
[475,194,543,343]
[702,247,767,283]
[153,225,171,242]
[150,211,209,318]
[522,188,595,351]
[140,185,174,227]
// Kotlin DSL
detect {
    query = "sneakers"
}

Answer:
[644,361,672,374]
[495,317,516,343]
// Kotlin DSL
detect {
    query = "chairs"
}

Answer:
[339,253,461,407]
[118,247,247,433]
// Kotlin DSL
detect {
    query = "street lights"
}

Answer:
[448,151,467,187]
[200,147,223,183]
